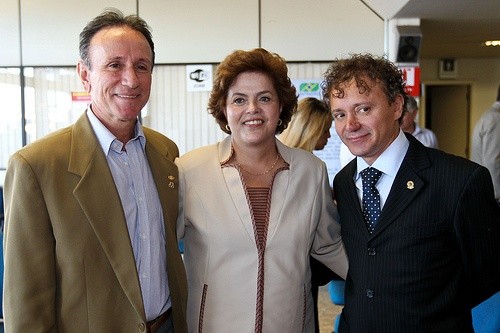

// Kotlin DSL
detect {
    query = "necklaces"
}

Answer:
[233,155,278,176]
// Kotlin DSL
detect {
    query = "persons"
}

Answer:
[174,48,349,333]
[398,94,437,149]
[472,85,500,202]
[2,7,188,333]
[310,52,500,333]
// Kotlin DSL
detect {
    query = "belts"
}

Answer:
[146,307,172,333]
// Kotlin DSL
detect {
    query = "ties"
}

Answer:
[360,167,382,236]
